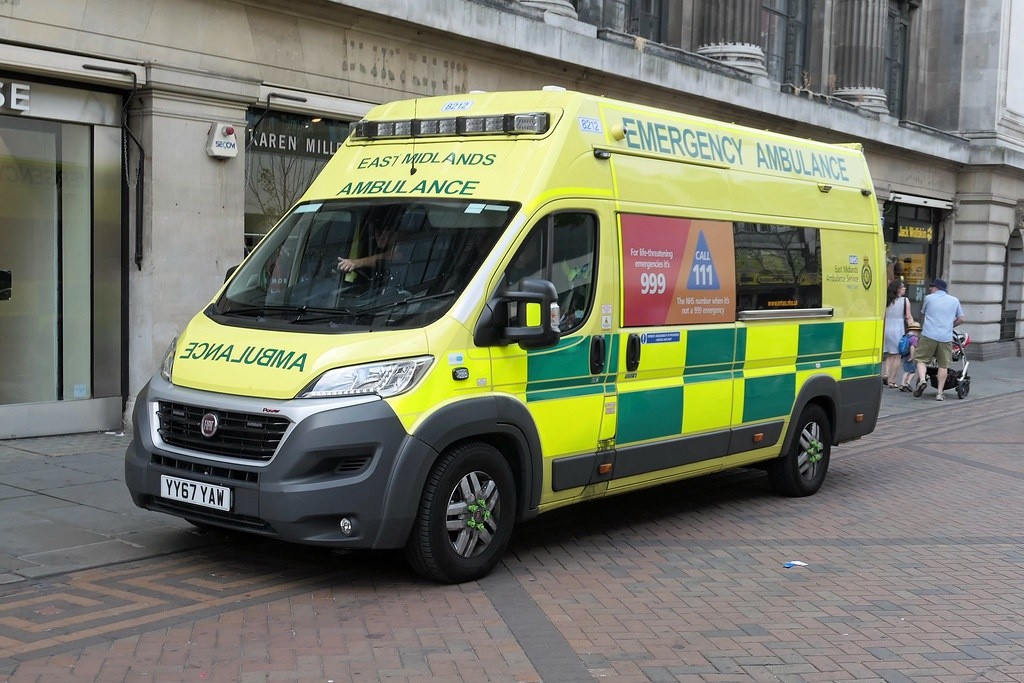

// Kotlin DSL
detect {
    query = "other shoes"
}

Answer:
[889,382,898,388]
[883,374,888,385]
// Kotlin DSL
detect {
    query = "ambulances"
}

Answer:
[124,83,886,586]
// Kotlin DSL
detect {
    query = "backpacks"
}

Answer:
[897,334,915,356]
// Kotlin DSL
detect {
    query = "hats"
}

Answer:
[929,279,948,293]
[908,322,923,330]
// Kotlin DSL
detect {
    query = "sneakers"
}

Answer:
[903,383,912,392]
[935,393,946,401]
[913,379,928,397]
[898,385,903,392]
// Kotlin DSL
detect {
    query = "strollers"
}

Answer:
[913,330,972,398]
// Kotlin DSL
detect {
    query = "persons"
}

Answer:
[338,205,409,272]
[912,279,965,400]
[899,322,922,392]
[559,257,586,331]
[883,279,913,388]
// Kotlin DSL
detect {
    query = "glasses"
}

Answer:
[900,286,905,288]
